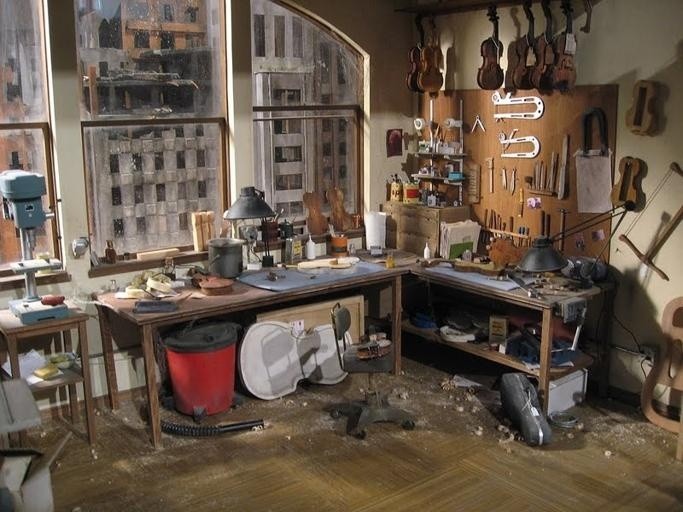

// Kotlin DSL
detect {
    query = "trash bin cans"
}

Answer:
[162,318,244,423]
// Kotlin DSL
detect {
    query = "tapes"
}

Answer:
[414,118,425,130]
[445,118,455,129]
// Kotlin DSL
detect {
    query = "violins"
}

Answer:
[406,9,443,92]
[477,0,577,97]
[187,265,234,295]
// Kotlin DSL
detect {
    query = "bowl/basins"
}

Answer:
[50,352,76,370]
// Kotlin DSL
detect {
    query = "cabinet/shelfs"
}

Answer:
[410,151,465,182]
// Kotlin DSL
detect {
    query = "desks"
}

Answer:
[89,251,411,453]
[356,247,618,425]
[0,297,99,451]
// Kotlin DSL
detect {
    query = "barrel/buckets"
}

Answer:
[207,238,247,278]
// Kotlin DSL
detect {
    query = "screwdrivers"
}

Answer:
[483,208,530,248]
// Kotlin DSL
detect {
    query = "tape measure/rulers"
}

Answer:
[484,158,494,193]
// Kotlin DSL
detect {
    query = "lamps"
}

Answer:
[222,186,278,267]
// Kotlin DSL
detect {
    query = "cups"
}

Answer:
[331,235,347,257]
[391,182,419,205]
[261,219,293,242]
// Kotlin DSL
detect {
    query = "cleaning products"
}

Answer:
[389,173,403,201]
[423,242,431,260]
[305,233,316,260]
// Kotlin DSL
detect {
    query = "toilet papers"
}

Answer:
[444,119,455,128]
[414,117,425,132]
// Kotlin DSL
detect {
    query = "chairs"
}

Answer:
[321,300,415,441]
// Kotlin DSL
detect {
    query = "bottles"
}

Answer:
[285,233,302,265]
[349,244,356,257]
[305,235,316,259]
[423,242,430,259]
[386,255,395,268]
[428,194,436,206]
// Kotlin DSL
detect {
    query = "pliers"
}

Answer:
[471,114,486,132]
